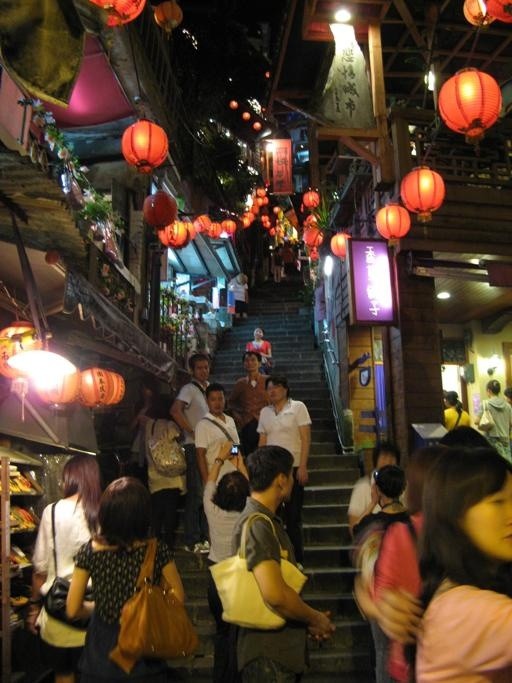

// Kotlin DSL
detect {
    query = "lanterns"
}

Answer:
[142,184,352,264]
[486,0,512,25]
[0,320,50,424]
[436,65,505,145]
[242,111,251,120]
[154,0,183,39]
[77,364,112,416]
[463,0,497,28]
[400,164,447,224]
[88,0,146,25]
[34,361,83,414]
[374,200,411,245]
[104,369,127,408]
[252,121,262,130]
[265,71,271,78]
[229,99,238,109]
[120,117,169,178]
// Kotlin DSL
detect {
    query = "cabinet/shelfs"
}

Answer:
[0,446,49,683]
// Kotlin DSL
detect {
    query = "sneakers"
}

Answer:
[183,542,210,554]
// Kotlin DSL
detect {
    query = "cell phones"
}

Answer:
[230,445,238,455]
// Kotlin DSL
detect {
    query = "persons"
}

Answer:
[64,474,186,682]
[194,381,250,489]
[169,353,212,556]
[221,346,272,460]
[255,374,314,571]
[246,326,272,375]
[118,371,190,555]
[344,378,510,680]
[229,445,339,683]
[203,438,251,683]
[26,453,106,682]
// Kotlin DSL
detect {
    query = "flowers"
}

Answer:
[31,95,200,337]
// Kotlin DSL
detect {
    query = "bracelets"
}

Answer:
[215,458,224,465]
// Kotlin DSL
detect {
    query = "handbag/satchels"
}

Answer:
[265,358,273,369]
[478,399,495,431]
[44,576,94,630]
[119,536,198,659]
[148,419,187,477]
[208,509,308,631]
